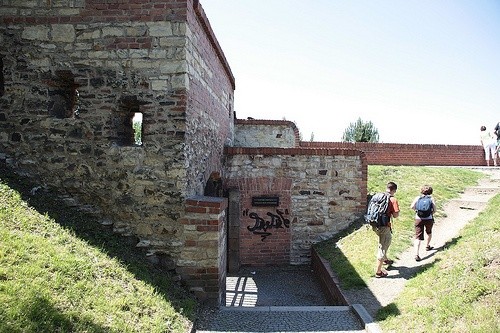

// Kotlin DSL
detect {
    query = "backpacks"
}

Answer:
[416,196,432,218]
[365,192,394,229]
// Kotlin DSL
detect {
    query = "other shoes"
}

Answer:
[426,245,430,251]
[416,255,420,261]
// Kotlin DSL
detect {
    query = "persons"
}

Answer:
[411,184,437,260]
[480,126,497,167]
[494,122,500,159]
[373,182,400,278]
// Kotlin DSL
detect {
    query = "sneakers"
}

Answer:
[376,271,388,277]
[382,259,393,264]
